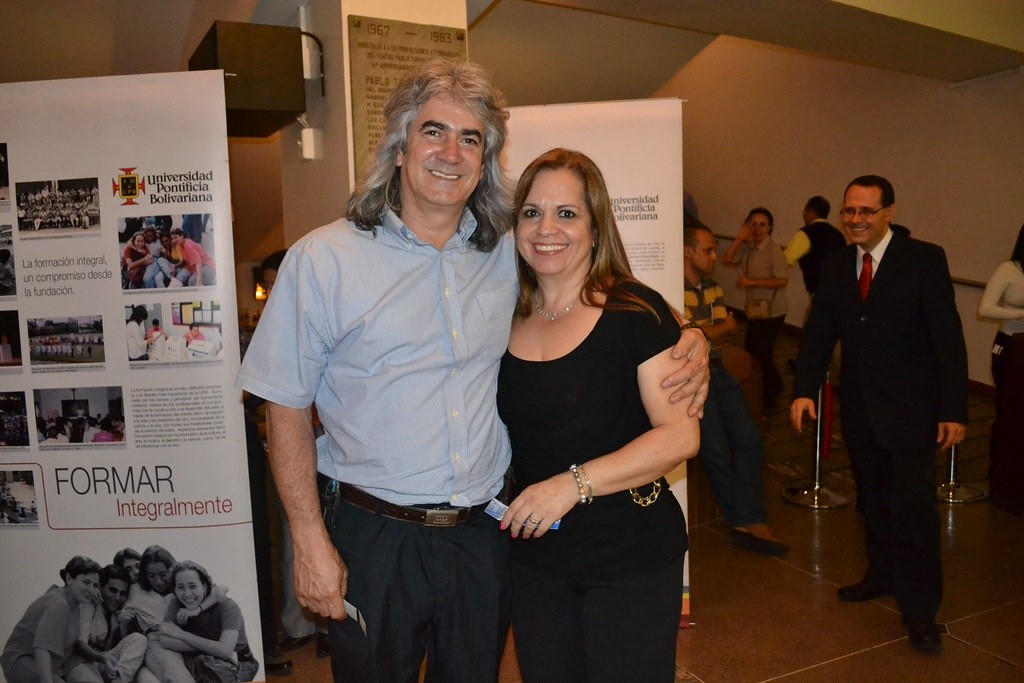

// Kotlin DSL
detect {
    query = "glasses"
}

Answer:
[839,207,886,218]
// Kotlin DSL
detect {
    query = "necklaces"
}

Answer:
[534,288,581,320]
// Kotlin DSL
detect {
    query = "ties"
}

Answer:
[858,253,873,306]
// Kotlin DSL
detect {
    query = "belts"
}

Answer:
[317,473,492,529]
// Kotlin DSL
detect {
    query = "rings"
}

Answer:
[528,516,540,525]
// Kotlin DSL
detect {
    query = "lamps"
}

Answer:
[251,248,286,301]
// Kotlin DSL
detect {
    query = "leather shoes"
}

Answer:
[315,632,330,658]
[280,635,313,651]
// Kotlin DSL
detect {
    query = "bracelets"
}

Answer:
[569,464,594,505]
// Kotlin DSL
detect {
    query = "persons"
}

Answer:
[730,195,847,397]
[0,549,259,683]
[240,61,522,683]
[121,214,215,288]
[29,332,103,359]
[16,186,100,231]
[978,223,1024,519]
[495,148,711,683]
[37,414,127,444]
[0,415,29,446]
[0,249,14,295]
[126,305,204,361]
[0,484,37,522]
[684,193,792,554]
[789,176,969,656]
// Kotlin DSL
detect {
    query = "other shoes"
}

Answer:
[731,525,789,559]
[837,570,896,602]
[262,641,293,675]
[900,609,940,655]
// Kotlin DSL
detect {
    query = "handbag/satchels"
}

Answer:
[746,299,770,319]
